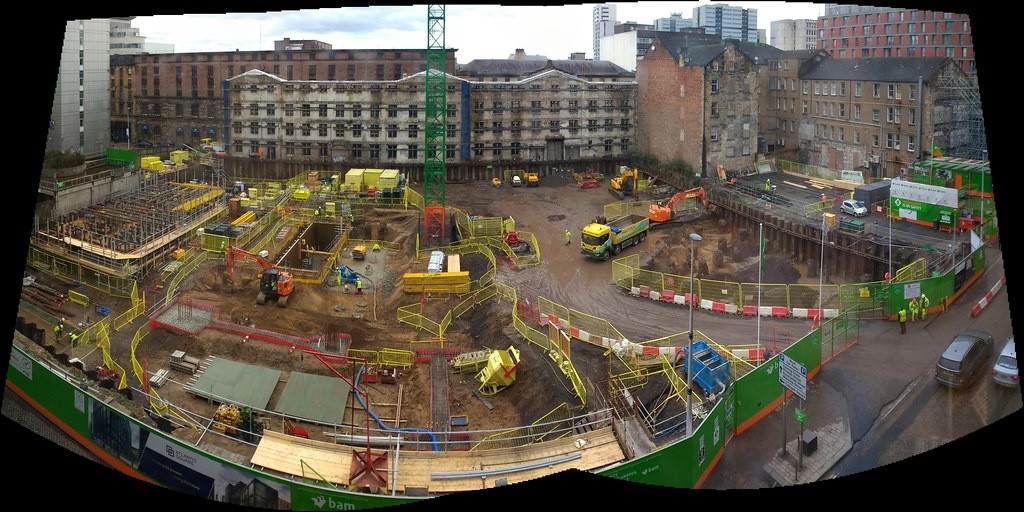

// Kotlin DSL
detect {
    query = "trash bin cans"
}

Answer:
[771,185,777,195]
[731,175,738,185]
[799,430,819,458]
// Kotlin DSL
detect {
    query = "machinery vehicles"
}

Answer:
[608,167,639,201]
[648,187,719,231]
[225,246,295,307]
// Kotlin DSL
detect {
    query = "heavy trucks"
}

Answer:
[581,213,649,261]
[521,173,539,187]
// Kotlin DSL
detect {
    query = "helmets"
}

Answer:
[62,318,65,321]
[921,294,926,298]
[60,325,64,328]
[69,332,72,335]
[912,299,917,302]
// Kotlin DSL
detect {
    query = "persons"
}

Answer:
[766,179,770,191]
[356,278,362,292]
[565,230,571,244]
[897,306,907,334]
[218,241,225,258]
[271,275,276,287]
[885,272,889,283]
[909,298,919,322]
[919,294,929,321]
[821,193,826,207]
[336,271,341,285]
[54,318,78,347]
[315,209,319,215]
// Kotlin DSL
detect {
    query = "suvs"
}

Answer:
[840,199,868,218]
[510,175,522,187]
[935,329,994,390]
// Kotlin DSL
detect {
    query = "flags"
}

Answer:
[933,145,944,157]
[760,231,765,272]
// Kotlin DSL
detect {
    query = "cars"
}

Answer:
[992,335,1020,387]
